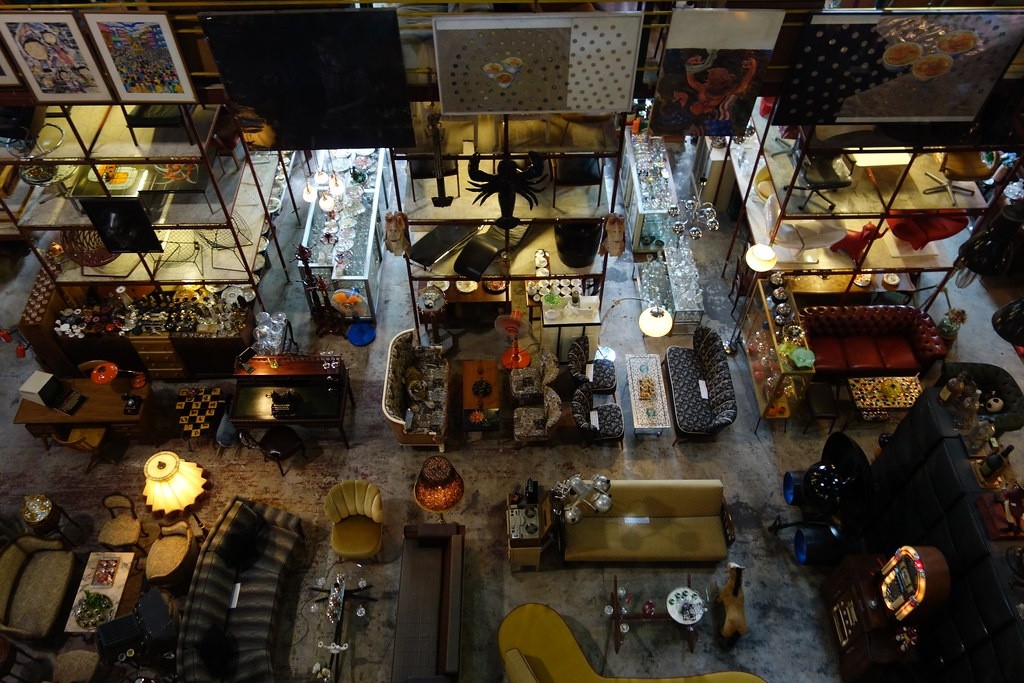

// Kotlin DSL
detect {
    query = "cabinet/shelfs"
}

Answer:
[820,388,1024,683]
[360,3,1024,436]
[0,2,411,383]
[225,354,357,450]
[507,492,542,572]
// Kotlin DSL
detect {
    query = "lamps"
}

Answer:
[141,451,209,539]
[414,455,464,523]
[724,244,776,355]
[92,362,147,387]
[595,298,673,361]
[296,150,343,211]
[667,137,720,240]
[557,475,613,524]
[0,327,35,359]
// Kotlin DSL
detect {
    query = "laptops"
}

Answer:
[38,375,88,416]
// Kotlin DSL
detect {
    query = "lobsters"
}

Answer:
[465,151,549,230]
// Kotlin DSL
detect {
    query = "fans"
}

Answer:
[494,310,533,370]
[331,286,376,347]
[418,285,454,354]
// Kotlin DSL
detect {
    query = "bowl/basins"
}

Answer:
[494,72,514,88]
[936,29,978,56]
[911,54,953,82]
[881,41,923,70]
[503,56,523,73]
[483,63,503,78]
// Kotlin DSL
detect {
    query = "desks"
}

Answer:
[0,636,44,683]
[13,378,159,448]
[850,146,912,168]
[281,320,297,354]
[23,495,79,548]
[176,387,226,453]
[300,565,378,683]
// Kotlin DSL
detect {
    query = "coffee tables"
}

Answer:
[462,359,501,446]
[837,376,925,434]
[63,552,135,642]
[625,353,671,437]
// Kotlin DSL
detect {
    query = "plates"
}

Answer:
[544,310,560,319]
[266,156,291,213]
[456,281,478,293]
[178,284,228,293]
[252,218,270,271]
[539,280,582,296]
[220,287,256,305]
[883,274,900,285]
[484,280,508,294]
[105,166,138,190]
[427,281,449,291]
[87,165,118,183]
[331,148,376,172]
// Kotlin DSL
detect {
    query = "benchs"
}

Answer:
[599,573,712,653]
[497,603,771,683]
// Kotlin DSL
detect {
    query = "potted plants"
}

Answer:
[936,307,967,351]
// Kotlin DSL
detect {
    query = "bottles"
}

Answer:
[955,390,982,427]
[938,371,967,408]
[979,444,1014,479]
[136,293,214,319]
[251,310,286,354]
[967,416,996,453]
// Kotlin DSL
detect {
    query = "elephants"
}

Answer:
[830,222,889,260]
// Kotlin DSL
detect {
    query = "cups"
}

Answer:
[533,295,540,301]
[692,590,699,601]
[682,589,689,599]
[76,609,103,627]
[674,591,681,602]
[667,597,676,606]
[640,376,655,398]
[640,254,673,309]
[528,281,537,296]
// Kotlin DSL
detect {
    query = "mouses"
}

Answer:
[128,399,134,406]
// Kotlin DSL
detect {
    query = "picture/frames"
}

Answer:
[0,10,114,104]
[0,44,24,87]
[79,10,200,105]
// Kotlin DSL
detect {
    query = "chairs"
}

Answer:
[728,241,769,315]
[920,148,1001,206]
[752,166,776,202]
[78,359,109,379]
[324,480,384,565]
[98,492,148,557]
[784,140,856,213]
[800,377,856,436]
[42,649,106,683]
[51,427,110,473]
[239,426,309,476]
[772,127,800,164]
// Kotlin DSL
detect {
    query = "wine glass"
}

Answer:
[19,275,55,325]
[216,320,243,337]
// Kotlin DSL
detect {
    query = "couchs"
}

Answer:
[883,210,971,259]
[569,335,617,403]
[799,304,948,383]
[765,190,848,263]
[382,328,453,454]
[667,325,738,446]
[560,479,736,565]
[934,362,1024,442]
[146,520,200,588]
[510,351,559,407]
[513,386,563,450]
[176,495,304,682]
[573,383,626,450]
[0,537,78,652]
[390,520,465,683]
